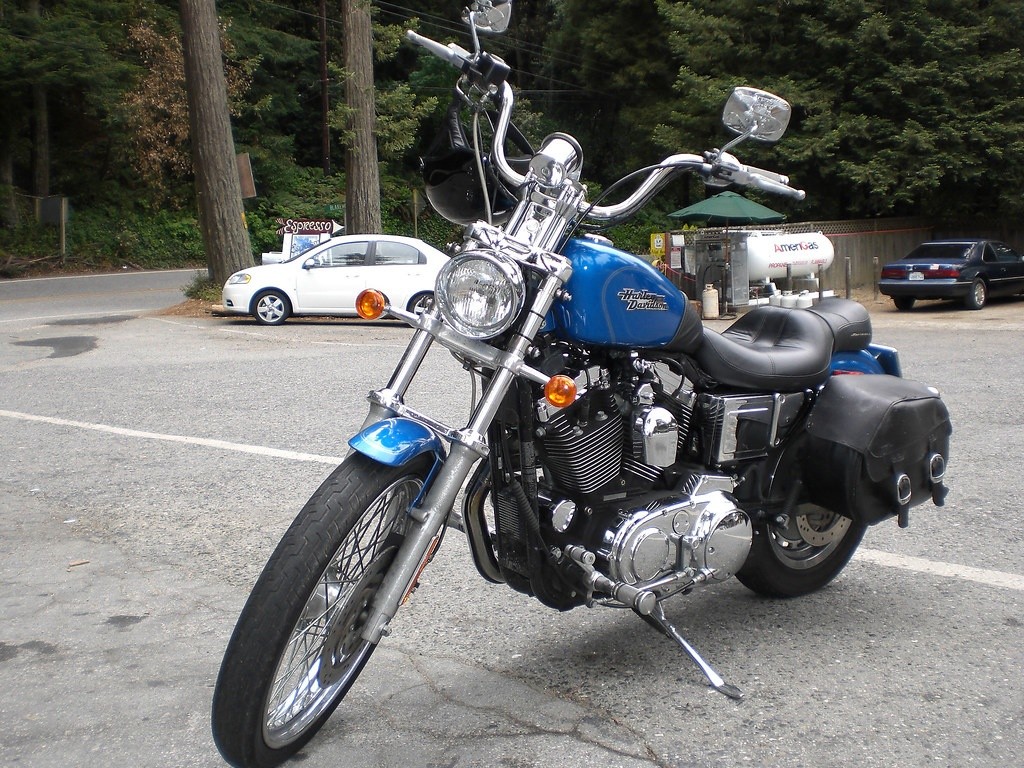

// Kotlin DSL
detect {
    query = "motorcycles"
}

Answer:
[203,0,950,768]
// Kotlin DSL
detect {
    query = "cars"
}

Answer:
[876,237,1024,311]
[220,232,494,328]
